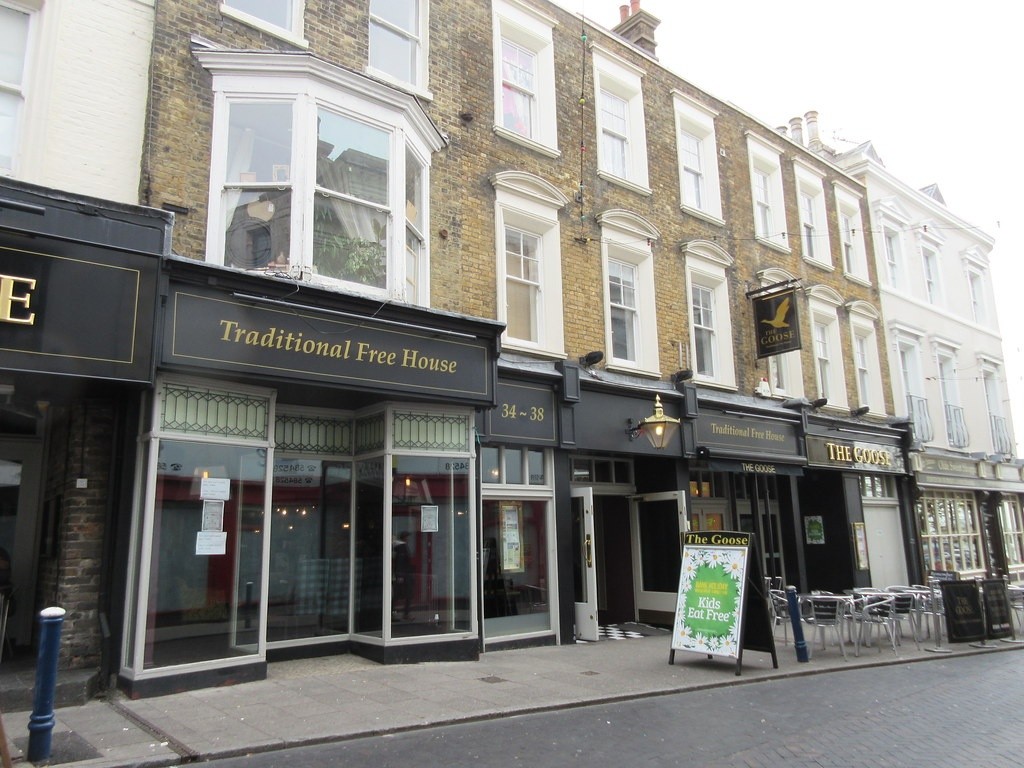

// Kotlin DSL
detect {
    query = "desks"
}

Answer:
[857,591,916,646]
[903,589,941,641]
[802,594,852,650]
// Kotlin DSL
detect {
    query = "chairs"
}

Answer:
[770,576,1024,662]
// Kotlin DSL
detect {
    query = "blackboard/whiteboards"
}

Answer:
[924,569,957,615]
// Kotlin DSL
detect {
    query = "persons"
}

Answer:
[392,531,419,622]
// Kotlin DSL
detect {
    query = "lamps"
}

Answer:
[625,397,679,448]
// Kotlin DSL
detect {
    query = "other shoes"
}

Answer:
[392,613,415,622]
